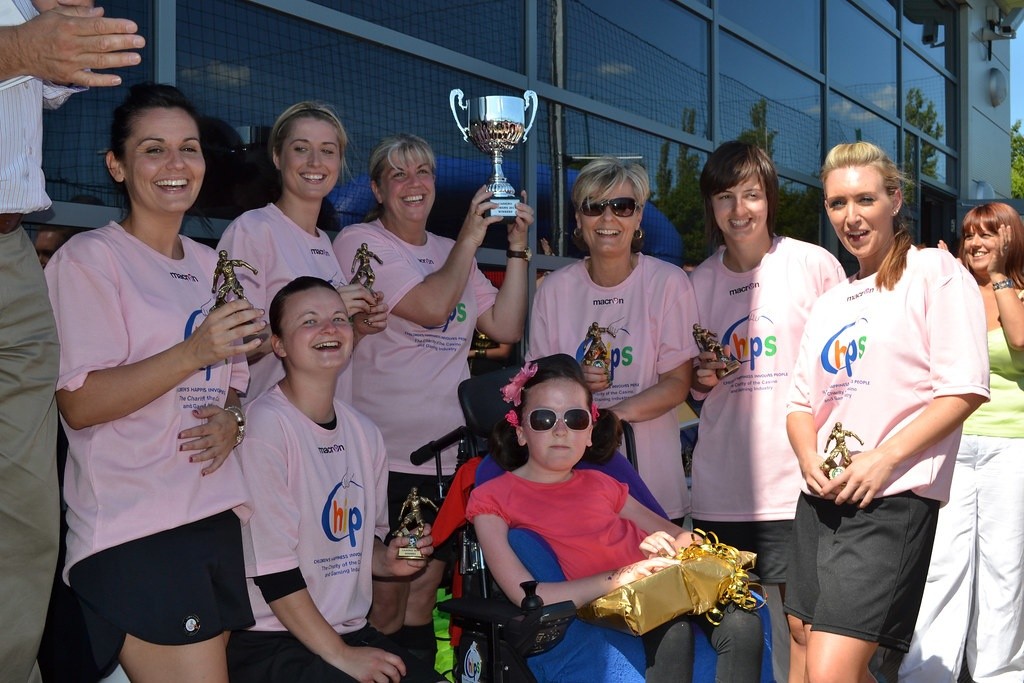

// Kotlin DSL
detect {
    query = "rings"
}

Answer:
[1003,247,1008,249]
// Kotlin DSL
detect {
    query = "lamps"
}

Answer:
[989,68,1007,108]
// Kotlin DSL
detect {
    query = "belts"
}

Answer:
[0,213,25,234]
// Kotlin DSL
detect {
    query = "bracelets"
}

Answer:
[225,406,246,431]
[476,349,486,360]
[993,280,1013,290]
[226,408,244,445]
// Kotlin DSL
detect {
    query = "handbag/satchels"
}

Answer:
[575,526,761,636]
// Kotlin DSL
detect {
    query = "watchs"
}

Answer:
[506,248,531,261]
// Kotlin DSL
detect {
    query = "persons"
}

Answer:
[469,271,514,361]
[689,138,847,683]
[466,353,762,682]
[214,100,387,409]
[36,194,102,266]
[526,157,698,524]
[781,142,991,683]
[1,1,148,683]
[896,203,1024,683]
[331,134,537,683]
[42,81,266,683]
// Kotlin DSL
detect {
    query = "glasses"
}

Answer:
[581,194,638,217]
[521,408,594,432]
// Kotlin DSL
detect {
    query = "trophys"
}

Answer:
[348,243,384,307]
[692,324,742,380]
[391,487,439,560]
[211,250,275,362]
[583,322,614,389]
[450,89,538,214]
[819,422,863,492]
